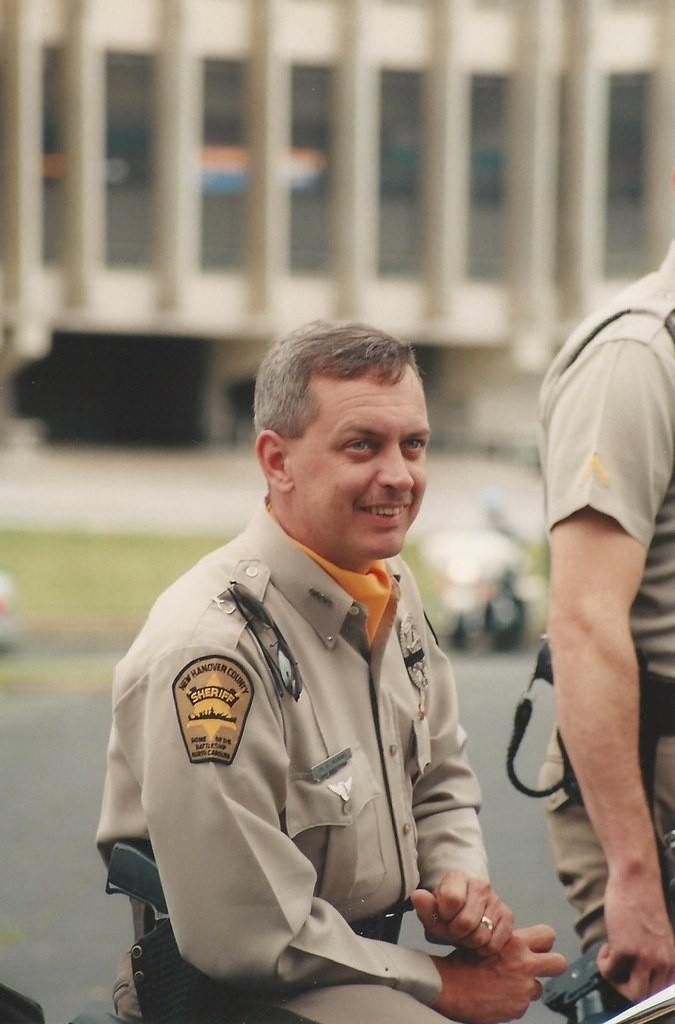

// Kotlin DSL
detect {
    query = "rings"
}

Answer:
[480,916,495,934]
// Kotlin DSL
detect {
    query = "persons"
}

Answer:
[537,236,675,1024]
[96,317,567,1024]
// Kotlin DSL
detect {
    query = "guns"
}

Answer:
[540,938,608,1024]
[104,841,170,931]
